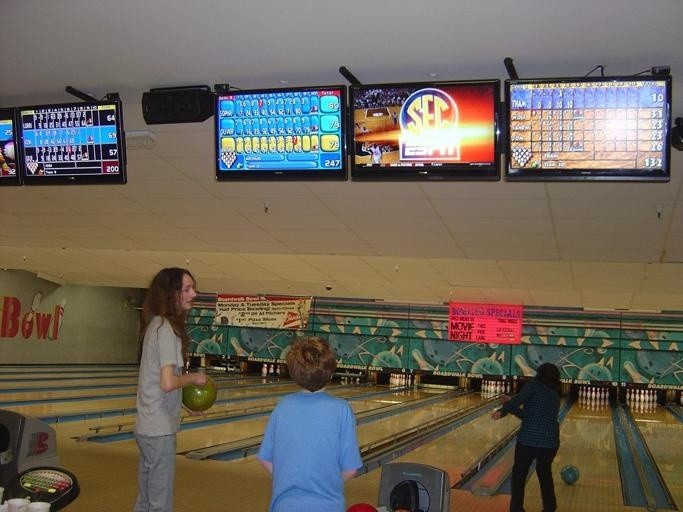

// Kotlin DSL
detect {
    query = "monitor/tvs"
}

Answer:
[0,106,21,185]
[506,73,673,184]
[349,78,500,185]
[215,84,346,182]
[18,100,126,185]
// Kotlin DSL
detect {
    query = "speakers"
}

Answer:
[142,91,215,125]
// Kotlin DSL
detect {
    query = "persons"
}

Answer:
[256,336,363,512]
[130,268,208,512]
[490,362,561,512]
